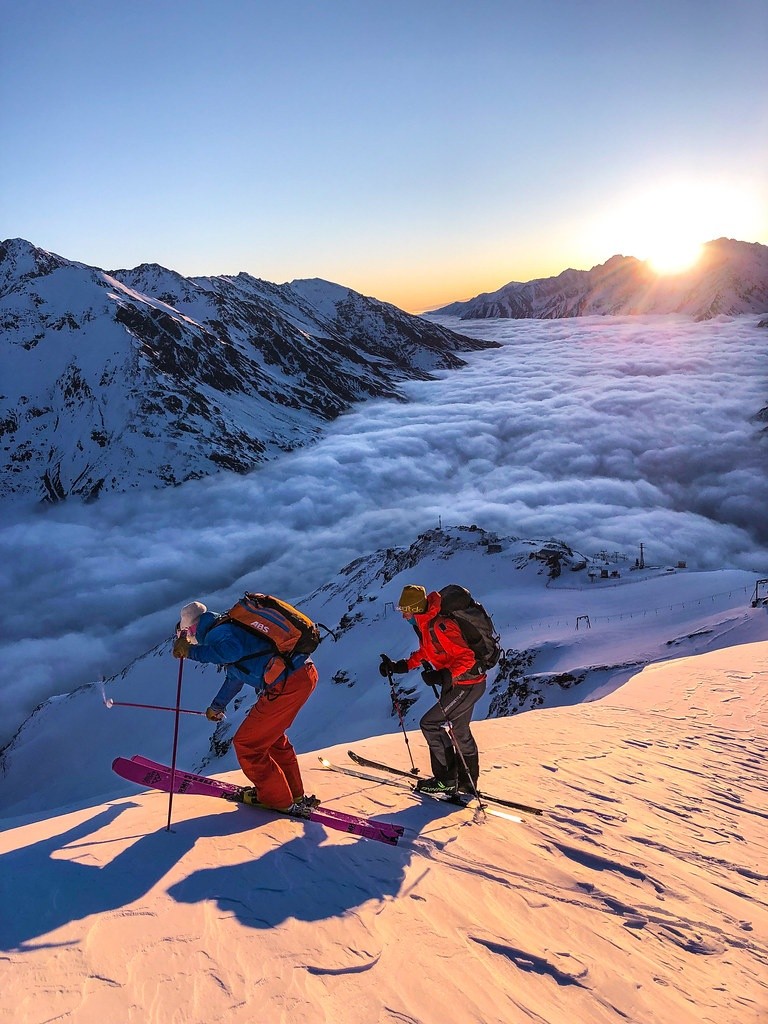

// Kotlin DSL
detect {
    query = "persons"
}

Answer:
[170,601,319,813]
[379,584,487,794]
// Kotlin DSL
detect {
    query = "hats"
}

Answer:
[398,583,427,613]
[180,601,207,630]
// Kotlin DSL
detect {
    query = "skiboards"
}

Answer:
[111,754,404,846]
[317,750,543,824]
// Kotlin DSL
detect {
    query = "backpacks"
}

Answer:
[209,590,337,701]
[413,584,505,681]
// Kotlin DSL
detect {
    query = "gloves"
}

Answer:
[173,636,190,659]
[379,659,408,677]
[206,706,224,721]
[421,667,452,686]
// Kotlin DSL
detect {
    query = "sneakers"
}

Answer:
[417,777,458,795]
[458,780,477,794]
[293,795,303,803]
[240,787,295,814]
